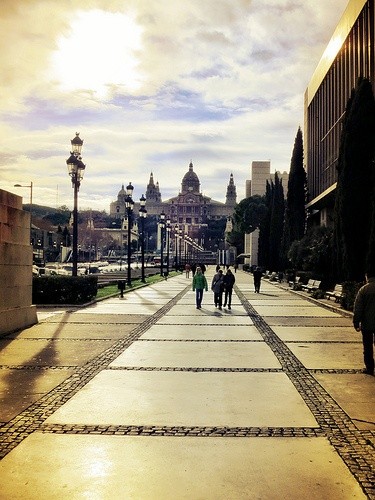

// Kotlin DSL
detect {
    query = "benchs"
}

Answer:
[288,276,301,289]
[325,284,345,303]
[301,279,322,298]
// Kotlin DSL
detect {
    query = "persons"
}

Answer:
[353,268,375,374]
[185,261,237,311]
[253,267,263,293]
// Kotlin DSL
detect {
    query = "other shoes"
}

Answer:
[215,303,217,307]
[228,304,231,309]
[360,368,374,374]
[199,305,201,308]
[224,303,226,307]
[197,306,199,309]
[219,306,222,310]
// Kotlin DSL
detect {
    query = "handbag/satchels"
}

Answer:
[211,283,216,289]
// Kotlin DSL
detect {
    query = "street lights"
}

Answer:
[166,215,171,276]
[157,210,165,277]
[66,130,86,277]
[183,234,204,265]
[138,192,147,283]
[13,182,34,214]
[123,182,133,291]
[178,230,182,273]
[173,223,179,272]
